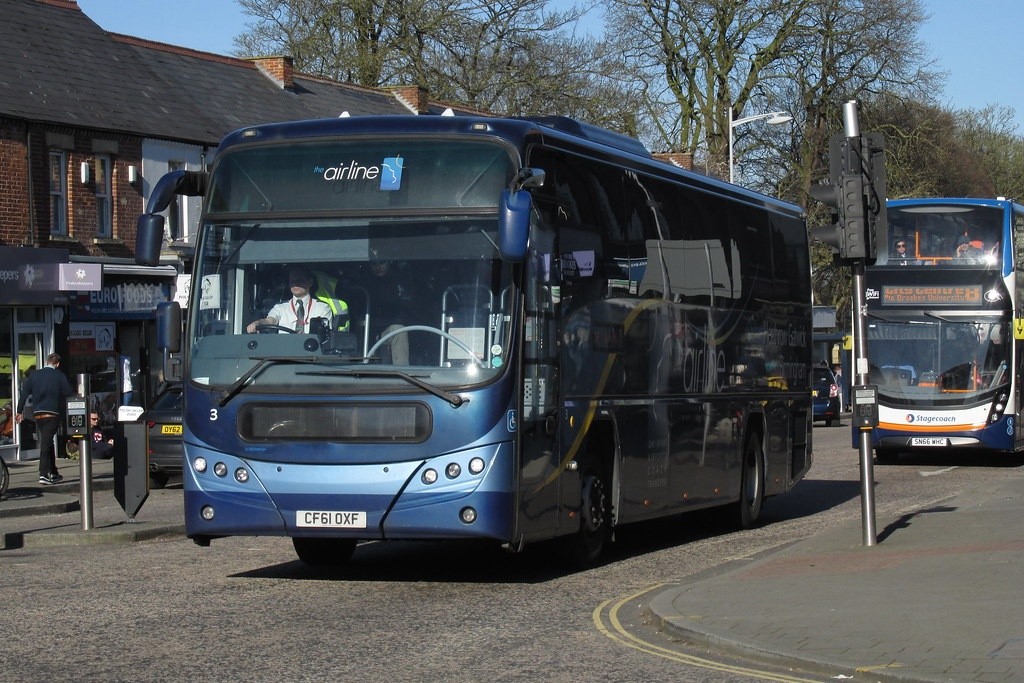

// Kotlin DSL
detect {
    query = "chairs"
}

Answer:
[871,365,916,386]
[911,241,999,265]
[256,263,349,334]
[557,278,807,350]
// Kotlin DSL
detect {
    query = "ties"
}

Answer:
[295,300,305,332]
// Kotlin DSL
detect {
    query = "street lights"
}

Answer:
[727,106,791,183]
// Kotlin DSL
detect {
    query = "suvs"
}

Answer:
[812,363,841,428]
[147,381,185,489]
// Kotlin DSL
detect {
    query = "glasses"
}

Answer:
[896,244,906,248]
[91,418,98,421]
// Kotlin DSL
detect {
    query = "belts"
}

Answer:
[35,415,57,419]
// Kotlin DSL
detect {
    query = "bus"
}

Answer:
[843,196,1024,454]
[134,113,815,568]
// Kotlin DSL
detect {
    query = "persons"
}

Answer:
[13,344,132,485]
[886,239,915,266]
[952,236,984,264]
[246,264,334,333]
[972,364,1006,388]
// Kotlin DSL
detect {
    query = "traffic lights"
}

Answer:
[805,179,874,264]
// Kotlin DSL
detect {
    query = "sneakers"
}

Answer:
[50,473,63,481]
[39,476,54,484]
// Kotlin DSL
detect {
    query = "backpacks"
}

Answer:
[66,441,79,460]
